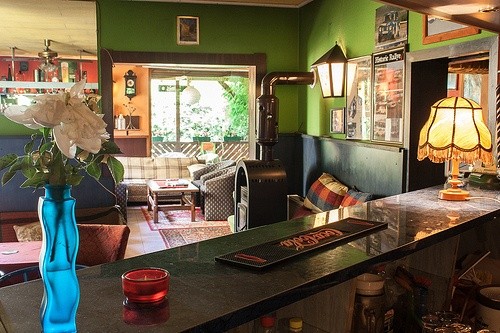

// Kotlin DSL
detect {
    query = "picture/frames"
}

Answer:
[176,16,200,46]
[422,15,481,45]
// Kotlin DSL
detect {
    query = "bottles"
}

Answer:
[287,318,303,333]
[258,316,276,333]
[467,172,500,192]
[115,114,125,130]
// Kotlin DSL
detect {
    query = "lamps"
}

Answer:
[35,39,58,73]
[181,75,201,105]
[311,40,347,99]
[416,96,492,200]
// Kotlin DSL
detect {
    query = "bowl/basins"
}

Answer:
[355,273,385,290]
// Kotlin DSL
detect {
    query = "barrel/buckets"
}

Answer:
[474,284,500,333]
[352,291,394,333]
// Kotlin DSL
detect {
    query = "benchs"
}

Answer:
[0,136,118,243]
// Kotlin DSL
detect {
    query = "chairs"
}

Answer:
[75,224,131,266]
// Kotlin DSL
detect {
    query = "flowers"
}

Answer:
[0,74,124,262]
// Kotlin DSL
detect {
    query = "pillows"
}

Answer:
[186,163,206,177]
[303,172,373,214]
[13,221,42,242]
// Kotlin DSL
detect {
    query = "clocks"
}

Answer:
[124,70,137,101]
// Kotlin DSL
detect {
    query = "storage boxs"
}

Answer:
[60,60,77,82]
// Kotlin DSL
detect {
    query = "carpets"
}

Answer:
[141,205,232,248]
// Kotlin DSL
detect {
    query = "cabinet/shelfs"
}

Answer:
[0,51,101,118]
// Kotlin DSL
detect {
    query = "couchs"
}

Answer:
[113,156,236,221]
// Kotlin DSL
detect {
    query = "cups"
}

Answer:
[121,266,170,303]
[123,298,170,324]
[421,310,491,333]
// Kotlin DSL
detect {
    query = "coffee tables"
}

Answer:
[145,179,199,223]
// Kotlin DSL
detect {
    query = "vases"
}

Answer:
[38,184,81,333]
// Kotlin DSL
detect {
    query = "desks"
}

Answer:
[114,135,150,156]
[0,242,44,288]
